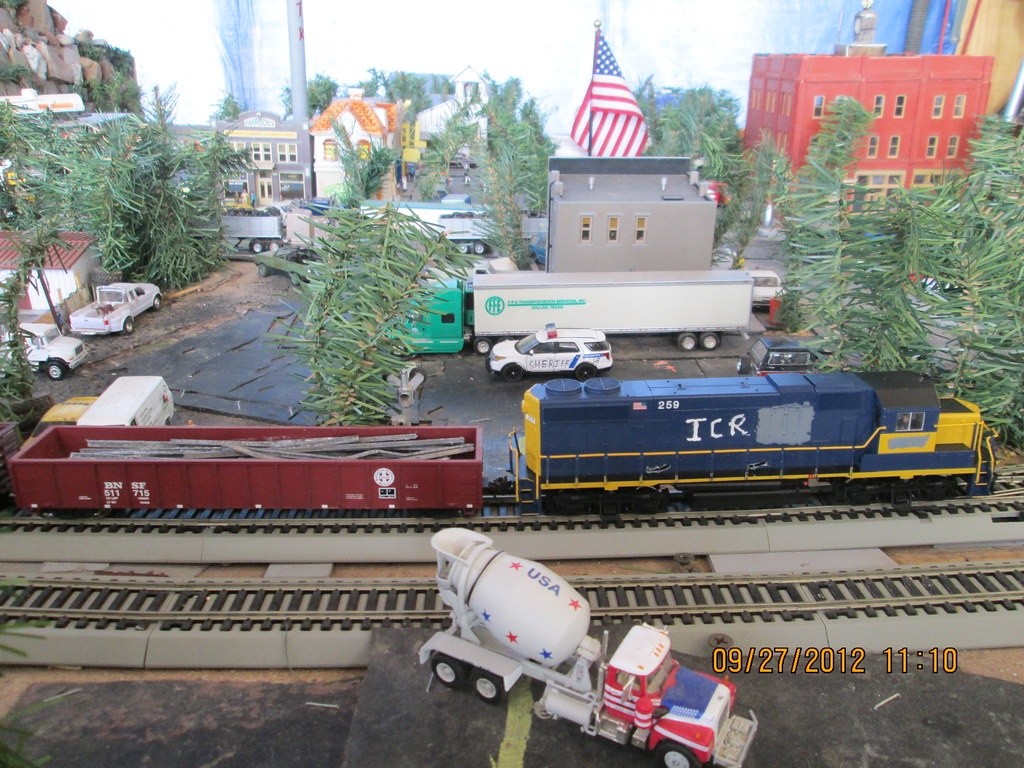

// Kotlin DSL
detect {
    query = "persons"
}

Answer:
[250,191,256,208]
[409,165,421,183]
[241,188,250,206]
[463,163,472,184]
[234,190,241,206]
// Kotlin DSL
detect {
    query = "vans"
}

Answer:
[76,376,174,426]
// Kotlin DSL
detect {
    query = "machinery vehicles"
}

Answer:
[419,527,759,768]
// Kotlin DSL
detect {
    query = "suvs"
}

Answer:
[485,323,614,383]
[736,336,870,376]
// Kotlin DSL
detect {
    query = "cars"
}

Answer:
[749,270,788,312]
[19,396,99,451]
[430,187,473,205]
[0,322,87,380]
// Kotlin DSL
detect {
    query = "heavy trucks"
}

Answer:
[379,268,755,357]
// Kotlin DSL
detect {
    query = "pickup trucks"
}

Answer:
[68,282,163,336]
[253,245,328,286]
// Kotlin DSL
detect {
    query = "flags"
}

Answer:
[570,29,648,157]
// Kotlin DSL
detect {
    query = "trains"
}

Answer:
[7,370,1000,519]
